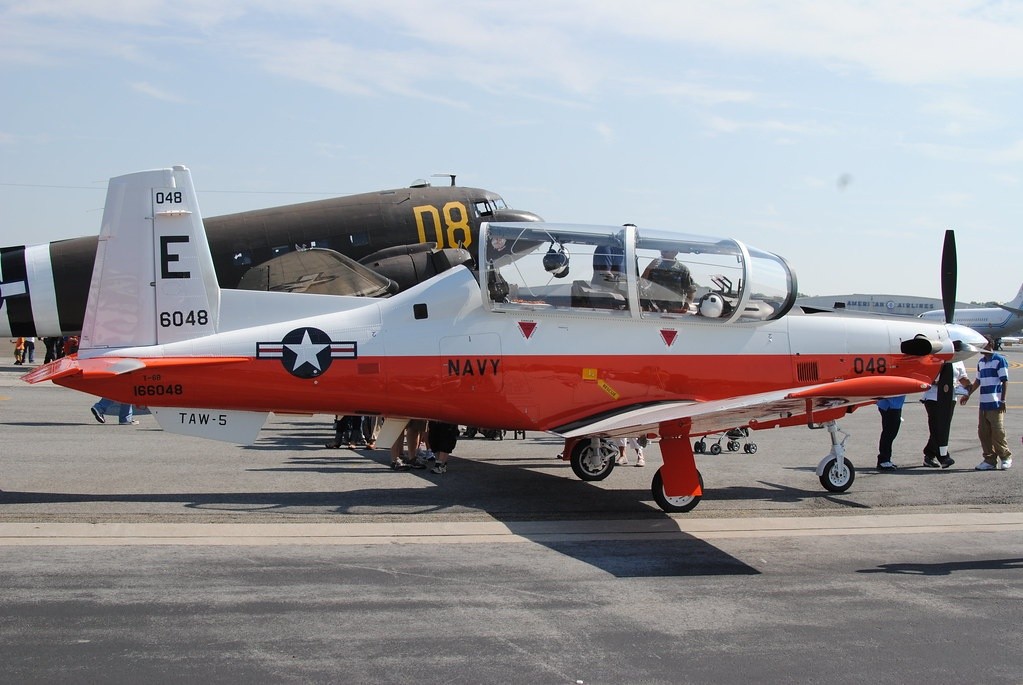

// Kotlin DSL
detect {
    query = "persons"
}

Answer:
[615,437,644,467]
[9,336,79,365]
[877,395,905,469]
[919,361,973,469]
[324,415,457,474]
[593,224,639,277]
[557,452,563,458]
[960,334,1012,471]
[91,397,138,425]
[640,249,696,314]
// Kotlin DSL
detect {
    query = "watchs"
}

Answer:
[1001,400,1006,403]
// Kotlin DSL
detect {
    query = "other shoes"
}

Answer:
[30,360,36,363]
[404,456,427,469]
[431,461,447,473]
[923,456,940,468]
[325,438,342,448]
[91,406,106,423]
[22,360,25,363]
[877,461,897,471]
[557,450,563,459]
[417,448,427,459]
[348,440,356,448]
[367,441,375,449]
[426,448,435,460]
[616,456,628,466]
[119,420,139,425]
[636,456,645,467]
[390,457,410,470]
[14,360,22,365]
[936,451,955,468]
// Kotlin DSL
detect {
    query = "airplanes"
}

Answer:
[0,173,546,338]
[18,166,989,514]
[917,283,1023,350]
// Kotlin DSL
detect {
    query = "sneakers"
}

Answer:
[1001,457,1012,469]
[976,461,999,470]
